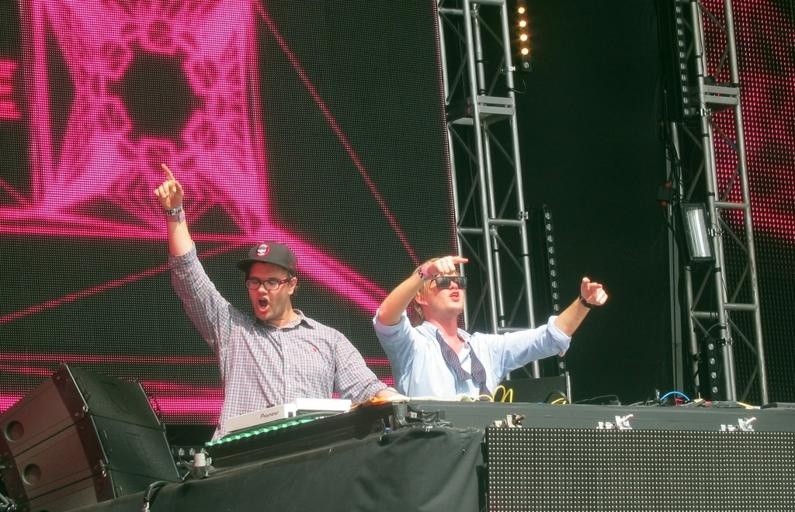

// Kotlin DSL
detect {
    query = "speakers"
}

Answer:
[0,364,184,512]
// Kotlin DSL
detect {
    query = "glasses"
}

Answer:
[245,278,289,290]
[429,277,467,289]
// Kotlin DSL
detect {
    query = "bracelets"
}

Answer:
[416,265,427,280]
[165,206,185,215]
[165,212,189,222]
[578,293,593,309]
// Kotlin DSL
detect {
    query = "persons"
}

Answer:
[372,251,611,402]
[150,158,410,441]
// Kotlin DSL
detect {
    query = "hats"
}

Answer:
[237,242,297,274]
